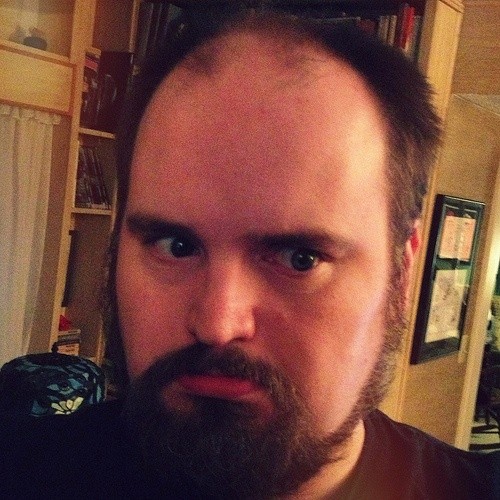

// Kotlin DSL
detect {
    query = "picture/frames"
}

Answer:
[408,194,486,367]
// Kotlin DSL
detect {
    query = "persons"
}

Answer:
[1,0,500,500]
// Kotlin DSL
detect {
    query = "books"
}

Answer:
[135,1,188,72]
[55,327,81,359]
[76,145,111,210]
[302,0,423,64]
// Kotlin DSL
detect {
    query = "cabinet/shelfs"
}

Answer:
[0,1,465,424]
